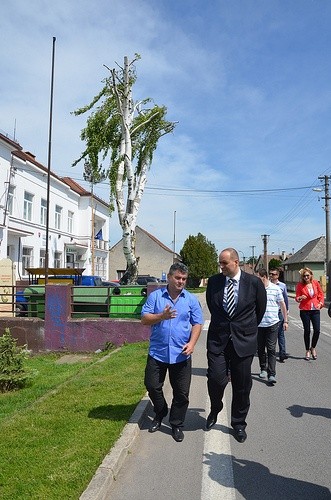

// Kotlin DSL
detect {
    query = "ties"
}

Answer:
[228,279,237,318]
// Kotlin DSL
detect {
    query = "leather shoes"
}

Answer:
[172,426,185,442]
[235,428,248,443]
[149,414,163,433]
[207,401,224,429]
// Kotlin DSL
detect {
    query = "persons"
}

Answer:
[141,262,204,442]
[256,269,288,383]
[268,268,288,363]
[206,248,267,443]
[295,267,324,360]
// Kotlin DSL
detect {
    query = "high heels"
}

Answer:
[306,351,311,361]
[310,346,318,360]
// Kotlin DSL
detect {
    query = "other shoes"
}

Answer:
[260,370,269,379]
[280,356,286,363]
[267,376,277,386]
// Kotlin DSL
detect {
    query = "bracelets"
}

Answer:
[320,302,323,307]
[283,321,288,324]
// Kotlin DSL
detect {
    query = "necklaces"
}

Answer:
[307,285,312,292]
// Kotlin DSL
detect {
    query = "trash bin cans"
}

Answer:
[109,288,147,318]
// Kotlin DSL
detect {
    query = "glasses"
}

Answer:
[303,274,311,277]
[270,273,279,276]
